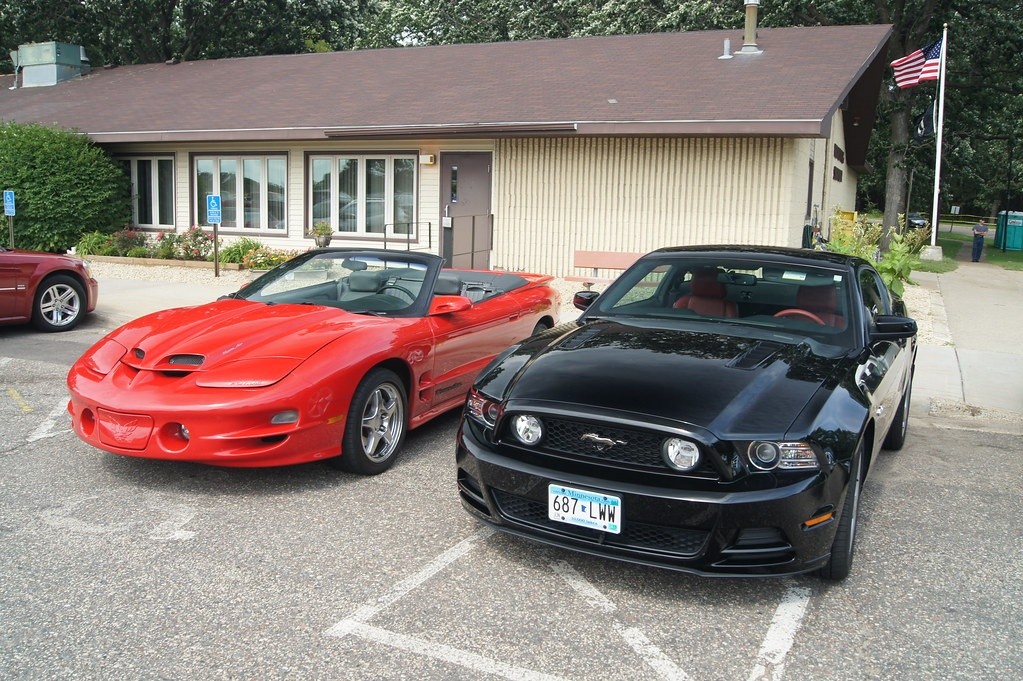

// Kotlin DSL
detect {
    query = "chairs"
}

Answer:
[673,268,739,319]
[788,282,845,329]
[341,271,383,301]
[433,277,463,296]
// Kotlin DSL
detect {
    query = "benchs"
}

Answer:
[563,250,670,291]
[336,276,504,304]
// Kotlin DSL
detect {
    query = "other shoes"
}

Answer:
[972,258,979,261]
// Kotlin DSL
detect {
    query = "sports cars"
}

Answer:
[67,249,557,476]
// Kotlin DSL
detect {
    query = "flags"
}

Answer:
[889,35,943,89]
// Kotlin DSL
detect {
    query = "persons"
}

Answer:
[972,218,989,262]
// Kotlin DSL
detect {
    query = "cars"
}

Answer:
[905,213,927,228]
[1,249,97,331]
[197,188,413,233]
[453,244,917,580]
